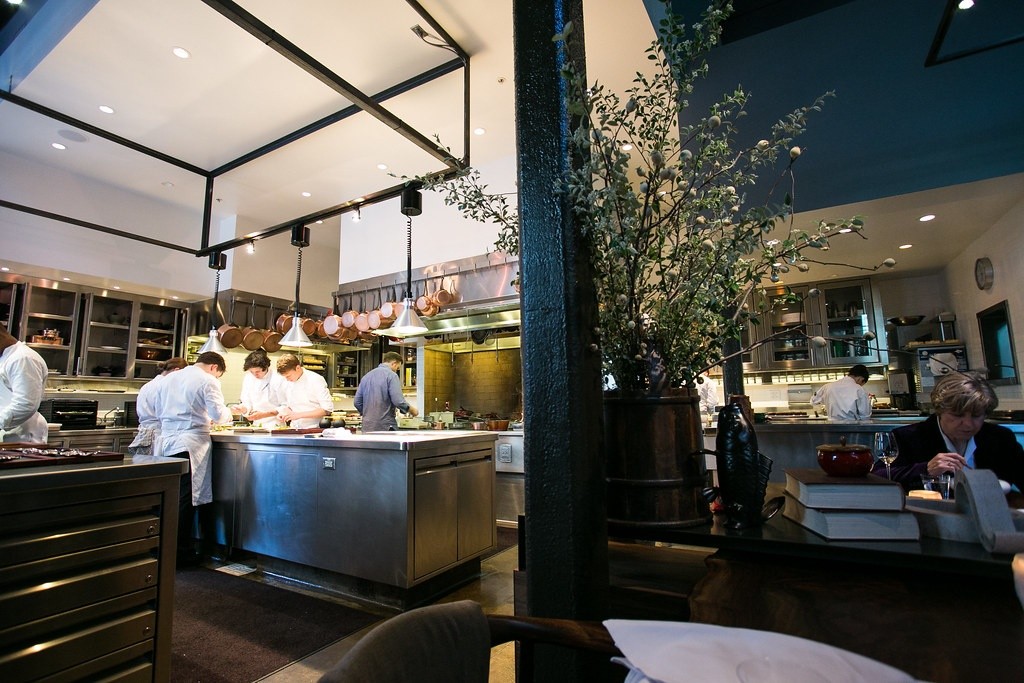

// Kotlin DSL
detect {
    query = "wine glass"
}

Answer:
[874,432,899,481]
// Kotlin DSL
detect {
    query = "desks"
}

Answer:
[514,481,1024,683]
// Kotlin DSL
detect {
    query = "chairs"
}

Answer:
[317,601,627,682]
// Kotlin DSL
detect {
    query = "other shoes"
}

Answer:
[208,550,226,562]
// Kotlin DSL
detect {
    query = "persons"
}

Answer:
[275,353,334,429]
[160,351,233,570]
[870,373,1024,497]
[127,356,189,456]
[0,321,48,444]
[692,369,717,414]
[354,352,419,431]
[810,365,873,420]
[231,351,286,428]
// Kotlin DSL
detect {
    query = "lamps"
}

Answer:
[276,225,314,348]
[197,253,229,355]
[389,190,429,336]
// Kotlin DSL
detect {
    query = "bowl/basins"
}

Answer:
[816,437,874,476]
[887,315,925,325]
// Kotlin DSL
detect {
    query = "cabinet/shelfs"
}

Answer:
[0,271,193,384]
[334,351,358,388]
[386,344,417,389]
[708,277,889,377]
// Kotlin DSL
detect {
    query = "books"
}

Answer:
[781,467,921,543]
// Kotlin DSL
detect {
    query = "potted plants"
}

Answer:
[387,0,985,542]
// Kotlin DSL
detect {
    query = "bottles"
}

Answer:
[871,397,877,408]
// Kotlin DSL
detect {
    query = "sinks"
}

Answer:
[106,426,127,428]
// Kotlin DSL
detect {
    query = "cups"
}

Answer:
[920,475,950,499]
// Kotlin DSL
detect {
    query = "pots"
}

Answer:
[431,422,445,430]
[488,420,509,431]
[219,277,457,352]
[472,422,484,429]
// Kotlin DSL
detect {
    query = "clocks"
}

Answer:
[975,256,993,290]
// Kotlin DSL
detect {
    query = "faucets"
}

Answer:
[104,406,120,421]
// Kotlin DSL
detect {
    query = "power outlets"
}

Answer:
[322,457,335,470]
[410,25,428,38]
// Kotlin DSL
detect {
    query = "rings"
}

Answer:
[948,461,951,466]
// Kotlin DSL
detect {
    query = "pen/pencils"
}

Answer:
[944,448,973,470]
